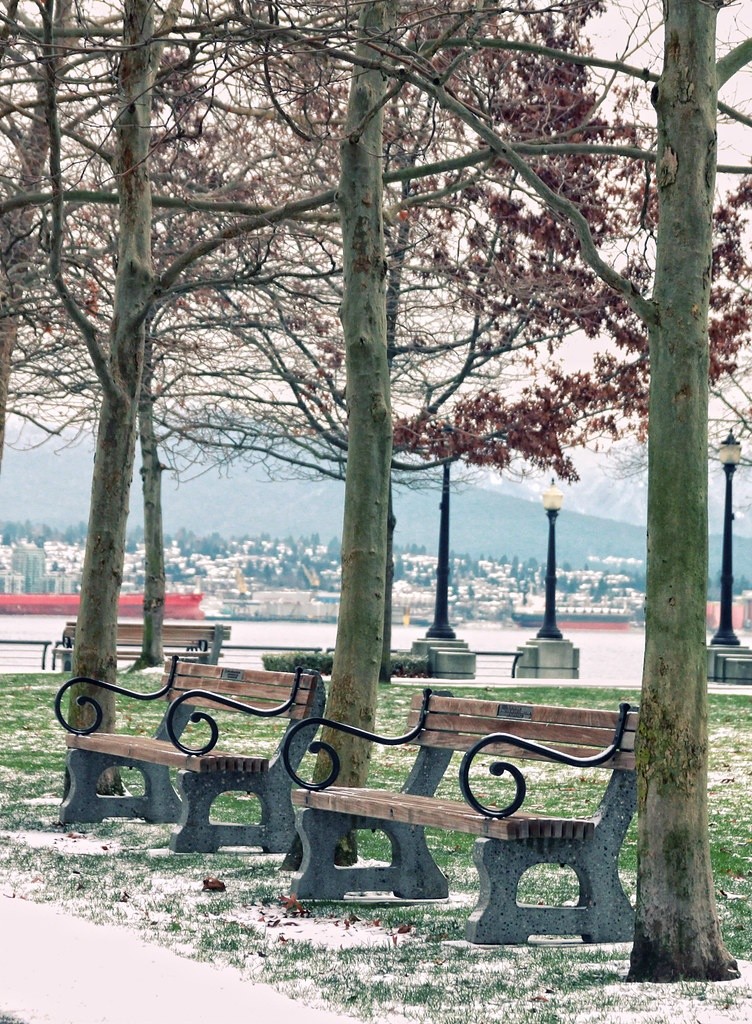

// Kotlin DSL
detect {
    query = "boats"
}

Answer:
[510,596,630,631]
[0,592,204,620]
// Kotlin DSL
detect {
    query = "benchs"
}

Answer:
[281,689,640,944]
[55,656,326,855]
[52,621,232,671]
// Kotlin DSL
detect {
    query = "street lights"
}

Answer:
[711,428,742,646]
[536,476,565,638]
